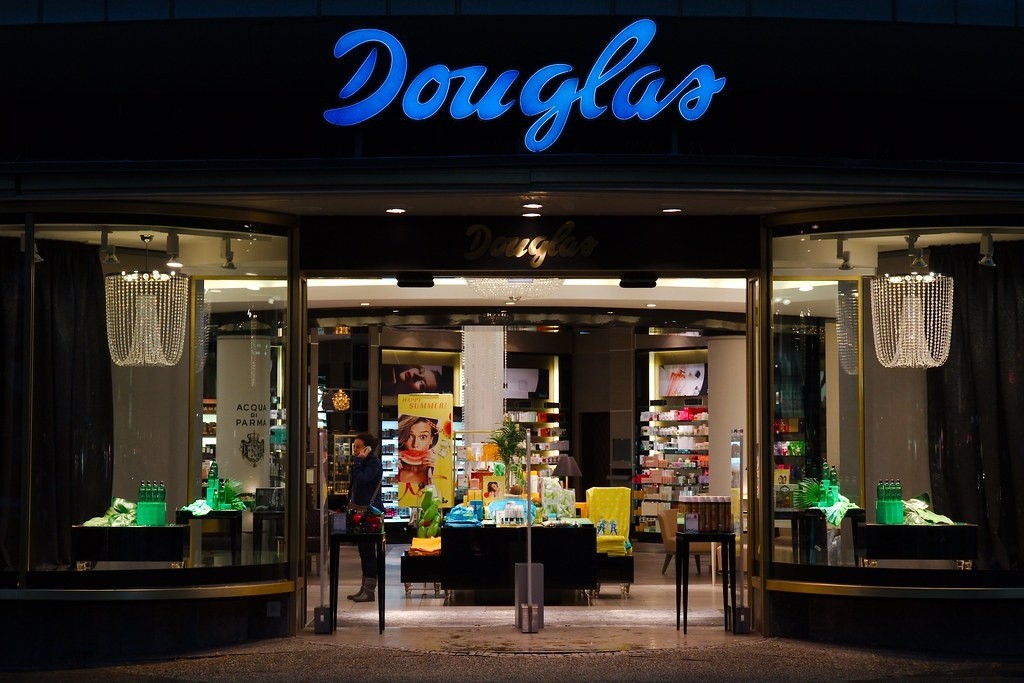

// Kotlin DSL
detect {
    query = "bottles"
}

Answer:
[678,496,732,533]
[139,479,166,502]
[877,478,902,501]
[207,458,231,510]
[818,459,838,507]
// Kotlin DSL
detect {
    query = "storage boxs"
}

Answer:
[137,502,166,525]
[463,489,483,519]
[207,479,220,509]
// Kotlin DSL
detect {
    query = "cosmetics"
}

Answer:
[457,409,570,526]
[202,421,225,510]
[637,406,710,534]
[380,428,410,518]
[139,480,166,502]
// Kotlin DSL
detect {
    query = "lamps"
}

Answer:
[221,237,237,269]
[166,234,182,266]
[551,457,582,491]
[979,233,996,266]
[20,232,44,262]
[99,230,120,263]
[105,234,189,367]
[870,272,954,368]
[837,281,860,376]
[904,234,927,268]
[837,238,855,271]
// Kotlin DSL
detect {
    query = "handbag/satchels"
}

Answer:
[345,503,383,535]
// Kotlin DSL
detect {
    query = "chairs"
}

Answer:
[658,509,721,574]
[307,510,335,574]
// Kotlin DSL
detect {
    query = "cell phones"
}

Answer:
[364,446,372,453]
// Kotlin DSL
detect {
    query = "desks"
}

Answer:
[676,508,978,635]
[330,526,634,635]
[70,510,284,567]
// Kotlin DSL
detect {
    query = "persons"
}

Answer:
[398,413,438,485]
[777,475,786,484]
[347,433,386,602]
[381,363,444,418]
[487,481,501,499]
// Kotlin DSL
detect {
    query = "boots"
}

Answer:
[347,578,377,601]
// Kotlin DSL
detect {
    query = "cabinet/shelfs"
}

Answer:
[202,398,805,544]
